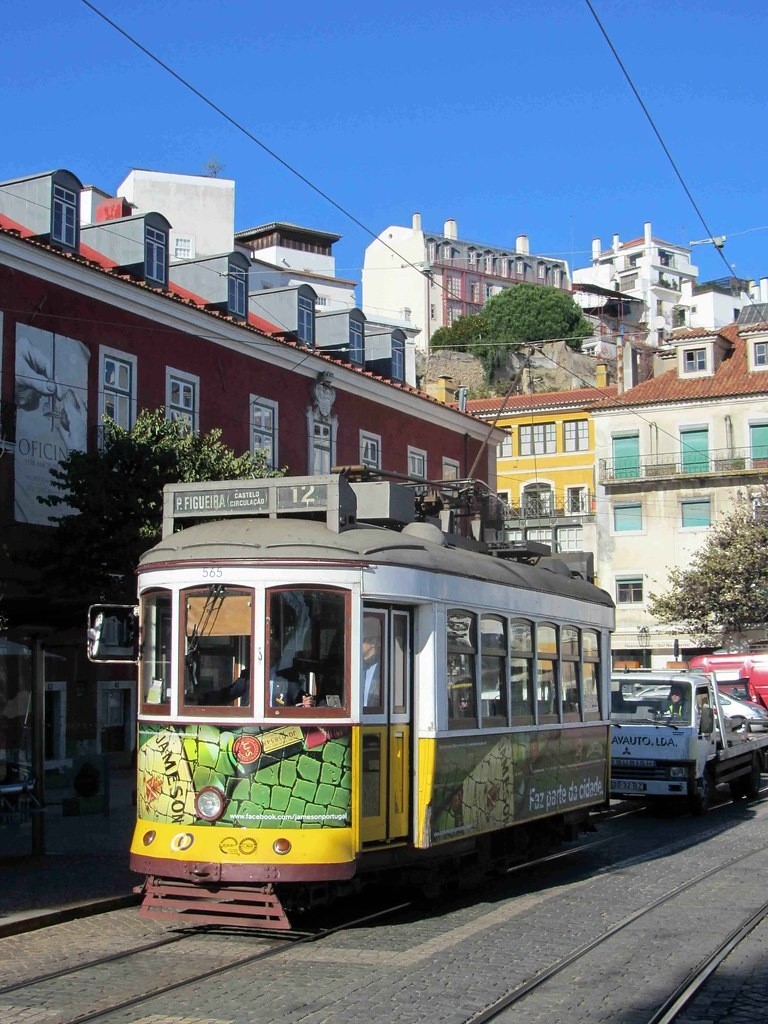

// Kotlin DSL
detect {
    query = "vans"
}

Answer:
[691,654,768,708]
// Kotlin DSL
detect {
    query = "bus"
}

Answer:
[87,347,619,938]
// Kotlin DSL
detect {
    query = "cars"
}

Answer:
[634,685,768,733]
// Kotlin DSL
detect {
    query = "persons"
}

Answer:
[185,638,313,707]
[363,624,380,707]
[655,687,690,720]
[456,696,475,718]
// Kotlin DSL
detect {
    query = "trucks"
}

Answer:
[601,669,768,817]
